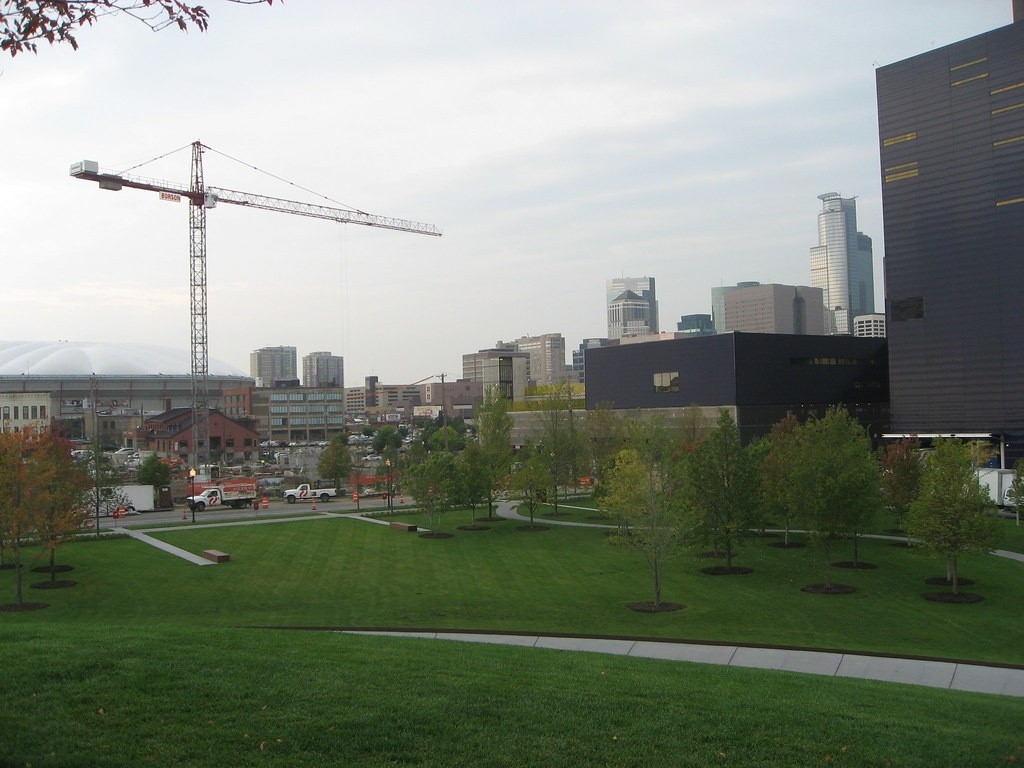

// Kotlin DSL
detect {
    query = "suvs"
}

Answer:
[123,459,143,470]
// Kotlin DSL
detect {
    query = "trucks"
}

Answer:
[962,467,1024,510]
[185,487,256,513]
[283,484,337,504]
[129,451,154,459]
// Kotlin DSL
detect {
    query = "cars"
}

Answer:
[259,438,329,447]
[113,447,135,456]
[347,433,420,461]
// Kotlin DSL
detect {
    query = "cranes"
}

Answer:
[69,140,444,481]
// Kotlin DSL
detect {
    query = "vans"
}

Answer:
[71,450,93,460]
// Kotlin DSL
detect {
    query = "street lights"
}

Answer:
[188,468,198,525]
[384,458,395,508]
